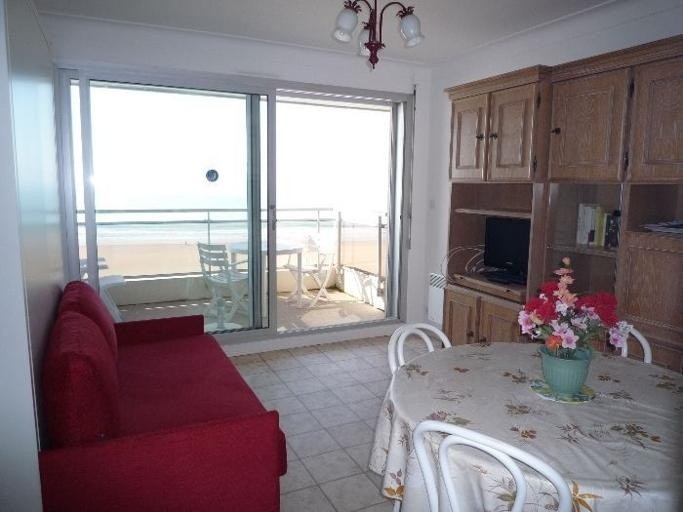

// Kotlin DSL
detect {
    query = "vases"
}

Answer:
[537,346,590,397]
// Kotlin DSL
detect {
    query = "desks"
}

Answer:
[368,341,683,512]
[230,245,302,308]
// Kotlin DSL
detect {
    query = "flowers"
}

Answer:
[518,256,633,358]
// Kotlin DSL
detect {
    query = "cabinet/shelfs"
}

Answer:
[442,284,528,350]
[624,34,683,184]
[548,47,630,181]
[443,65,551,183]
[616,238,682,373]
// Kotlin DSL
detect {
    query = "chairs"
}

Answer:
[283,252,336,308]
[412,420,572,511]
[387,322,453,376]
[609,326,652,364]
[197,243,249,323]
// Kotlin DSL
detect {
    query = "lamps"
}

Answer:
[333,1,426,70]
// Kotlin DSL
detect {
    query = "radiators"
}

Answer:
[427,272,446,326]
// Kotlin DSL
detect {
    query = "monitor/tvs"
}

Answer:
[479,216,531,285]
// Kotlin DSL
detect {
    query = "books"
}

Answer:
[641,218,683,234]
[576,202,620,252]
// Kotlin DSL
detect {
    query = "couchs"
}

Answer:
[38,280,287,512]
